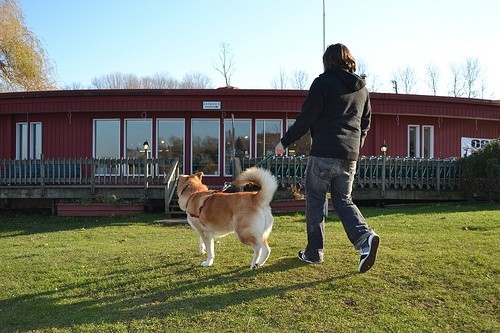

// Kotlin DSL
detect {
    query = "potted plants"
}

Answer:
[55,192,148,217]
[270,184,333,211]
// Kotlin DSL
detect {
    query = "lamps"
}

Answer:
[143,139,149,150]
[380,140,389,154]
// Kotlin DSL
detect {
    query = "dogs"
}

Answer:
[176,166,279,271]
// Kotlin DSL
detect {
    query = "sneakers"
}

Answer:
[297,250,324,264]
[358,232,380,273]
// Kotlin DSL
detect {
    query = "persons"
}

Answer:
[274,43,380,272]
